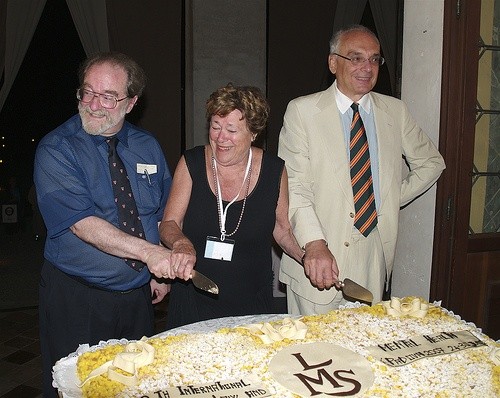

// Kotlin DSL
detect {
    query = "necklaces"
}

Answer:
[212,154,252,236]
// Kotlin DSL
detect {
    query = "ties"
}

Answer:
[348,102,378,237]
[104,134,147,271]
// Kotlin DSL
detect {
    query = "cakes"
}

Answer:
[75,296,500,398]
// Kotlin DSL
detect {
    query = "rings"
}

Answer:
[163,272,167,276]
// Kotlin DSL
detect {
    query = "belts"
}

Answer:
[72,276,151,294]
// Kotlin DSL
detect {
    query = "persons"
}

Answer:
[33,51,176,398]
[159,83,338,331]
[278,24,446,316]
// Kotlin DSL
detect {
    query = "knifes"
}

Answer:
[334,278,374,304]
[190,270,219,294]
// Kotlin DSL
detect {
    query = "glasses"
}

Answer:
[333,52,383,65]
[76,87,130,109]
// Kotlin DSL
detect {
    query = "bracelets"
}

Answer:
[301,254,305,266]
